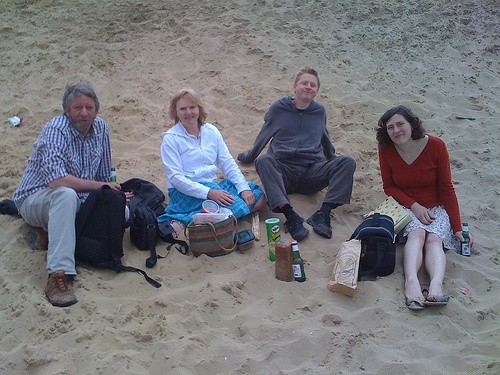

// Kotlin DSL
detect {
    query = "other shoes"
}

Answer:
[424,285,450,306]
[405,293,426,310]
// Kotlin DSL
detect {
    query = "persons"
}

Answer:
[375,105,475,310]
[159,88,266,242]
[14,82,134,307]
[237,66,357,242]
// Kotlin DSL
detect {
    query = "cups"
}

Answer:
[201,200,220,213]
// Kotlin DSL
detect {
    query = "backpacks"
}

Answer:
[74,184,127,274]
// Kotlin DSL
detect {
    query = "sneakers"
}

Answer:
[31,226,48,251]
[44,270,78,307]
[284,213,309,242]
[306,210,333,240]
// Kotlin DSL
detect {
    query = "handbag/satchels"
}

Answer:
[347,212,396,282]
[184,207,239,257]
[121,177,166,214]
[129,206,162,268]
[362,195,412,234]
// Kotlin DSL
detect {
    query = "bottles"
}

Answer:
[460,223,470,257]
[110,167,116,184]
[291,241,306,283]
